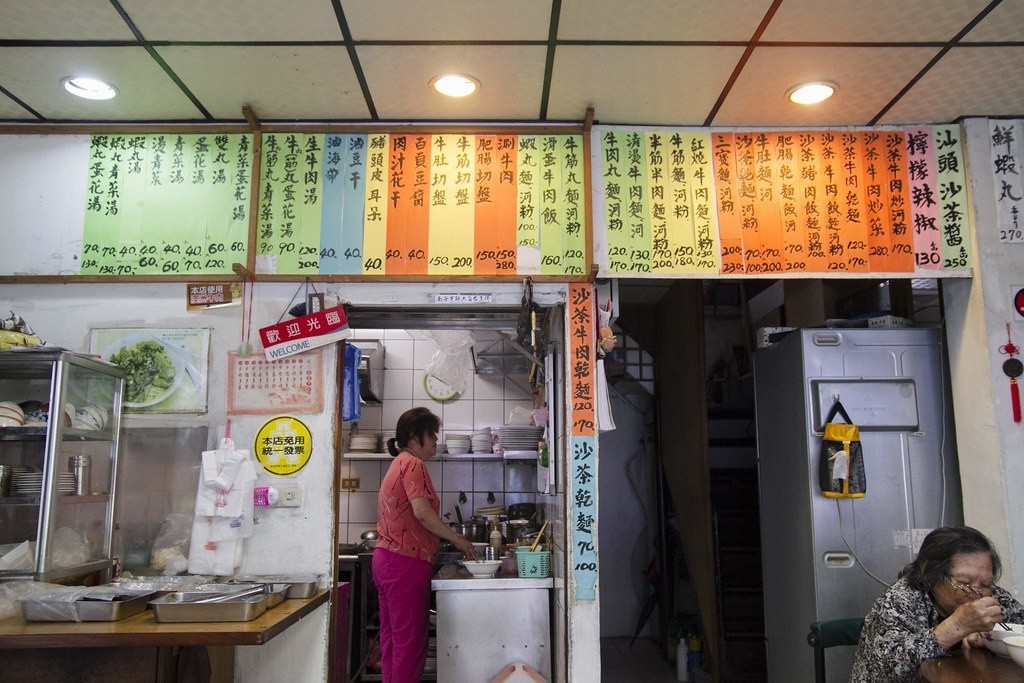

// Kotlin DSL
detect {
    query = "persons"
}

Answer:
[847,525,1024,683]
[372,408,474,683]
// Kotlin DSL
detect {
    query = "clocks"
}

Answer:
[424,372,458,402]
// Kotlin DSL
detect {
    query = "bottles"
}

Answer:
[490,526,502,556]
[480,516,490,543]
[68,455,92,496]
[500,552,518,578]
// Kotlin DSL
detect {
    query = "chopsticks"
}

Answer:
[469,546,486,563]
[960,585,1011,631]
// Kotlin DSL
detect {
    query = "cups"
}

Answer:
[486,547,500,578]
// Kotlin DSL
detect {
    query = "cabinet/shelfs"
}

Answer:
[1,349,126,587]
[655,280,915,683]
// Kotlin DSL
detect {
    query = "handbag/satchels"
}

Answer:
[819,401,868,498]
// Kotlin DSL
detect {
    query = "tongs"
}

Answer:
[181,583,274,609]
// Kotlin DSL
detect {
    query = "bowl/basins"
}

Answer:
[461,559,503,578]
[0,398,108,432]
[980,623,1024,657]
[446,434,471,454]
[476,505,507,539]
[436,443,446,454]
[1002,636,1024,671]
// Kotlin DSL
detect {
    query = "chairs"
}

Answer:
[807,617,867,683]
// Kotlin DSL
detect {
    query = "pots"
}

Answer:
[449,517,485,543]
[507,523,540,544]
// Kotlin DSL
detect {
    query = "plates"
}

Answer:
[471,427,492,453]
[349,434,400,453]
[98,337,185,407]
[10,465,76,497]
[499,425,545,450]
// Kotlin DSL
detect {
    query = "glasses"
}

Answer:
[945,576,994,604]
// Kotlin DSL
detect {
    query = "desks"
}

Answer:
[0,591,329,683]
[920,647,1024,683]
